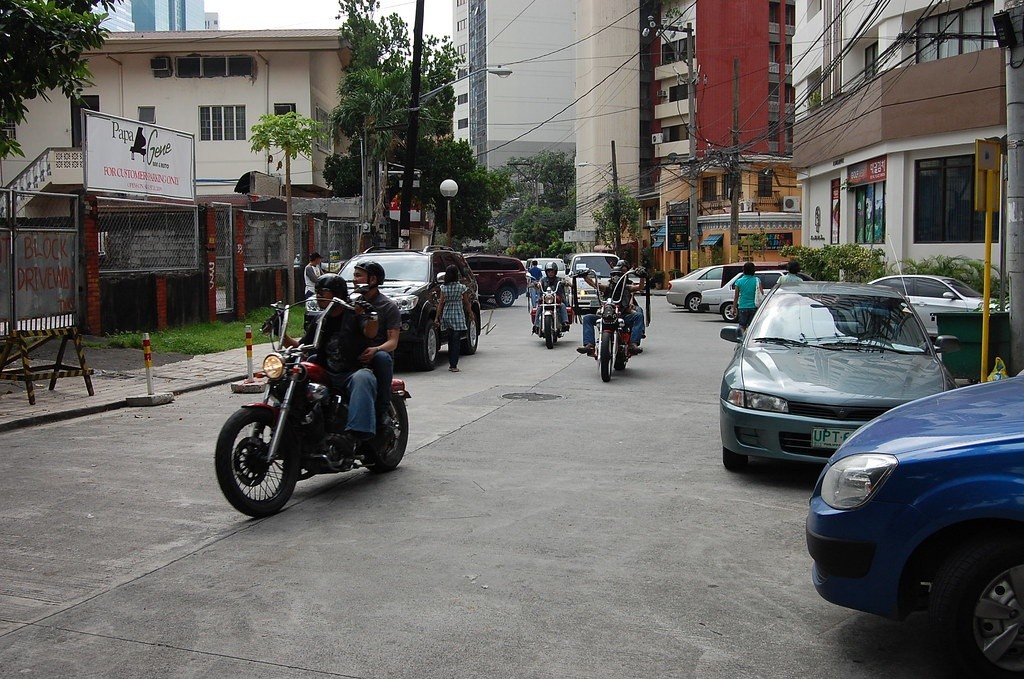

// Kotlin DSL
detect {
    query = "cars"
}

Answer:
[564,253,622,315]
[699,269,816,323]
[830,274,1011,338]
[804,372,1024,679]
[666,265,723,313]
[526,258,569,278]
[718,281,959,473]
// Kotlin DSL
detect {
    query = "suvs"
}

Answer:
[303,244,482,371]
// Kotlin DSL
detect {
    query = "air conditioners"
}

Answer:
[739,200,752,211]
[646,220,658,226]
[150,58,170,71]
[651,133,663,144]
[656,90,668,97]
[780,195,800,212]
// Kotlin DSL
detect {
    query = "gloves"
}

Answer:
[353,300,378,322]
[561,280,571,287]
[533,281,540,289]
[579,269,587,278]
[261,312,288,338]
[635,266,648,278]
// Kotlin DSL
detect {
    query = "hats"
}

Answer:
[309,251,324,261]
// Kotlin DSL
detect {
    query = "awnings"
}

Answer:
[652,225,668,247]
[594,240,637,250]
[700,233,724,247]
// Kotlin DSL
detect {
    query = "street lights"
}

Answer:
[578,161,622,260]
[440,179,458,248]
[398,67,513,249]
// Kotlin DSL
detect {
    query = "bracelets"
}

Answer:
[376,346,380,352]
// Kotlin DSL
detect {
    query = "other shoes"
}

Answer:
[562,323,570,331]
[577,343,595,355]
[627,343,643,355]
[532,326,537,332]
[375,411,392,440]
[448,367,463,372]
[326,432,354,458]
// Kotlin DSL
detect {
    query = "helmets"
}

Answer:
[545,261,558,275]
[616,260,630,266]
[315,273,349,300]
[354,260,386,286]
[610,266,622,275]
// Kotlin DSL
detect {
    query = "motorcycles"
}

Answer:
[214,283,413,520]
[570,267,647,382]
[529,280,574,349]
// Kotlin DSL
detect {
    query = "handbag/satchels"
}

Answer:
[755,276,764,308]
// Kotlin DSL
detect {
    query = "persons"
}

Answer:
[534,262,570,334]
[777,260,802,285]
[577,260,648,356]
[733,263,764,336]
[305,253,323,299]
[529,261,542,308]
[267,259,402,452]
[434,265,474,371]
